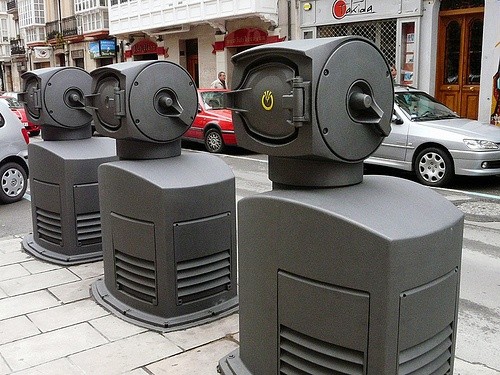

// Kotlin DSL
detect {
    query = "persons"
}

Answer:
[209,71,228,90]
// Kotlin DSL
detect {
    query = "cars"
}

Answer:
[182,88,239,151]
[0,93,41,137]
[362,83,500,186]
[0,98,32,204]
